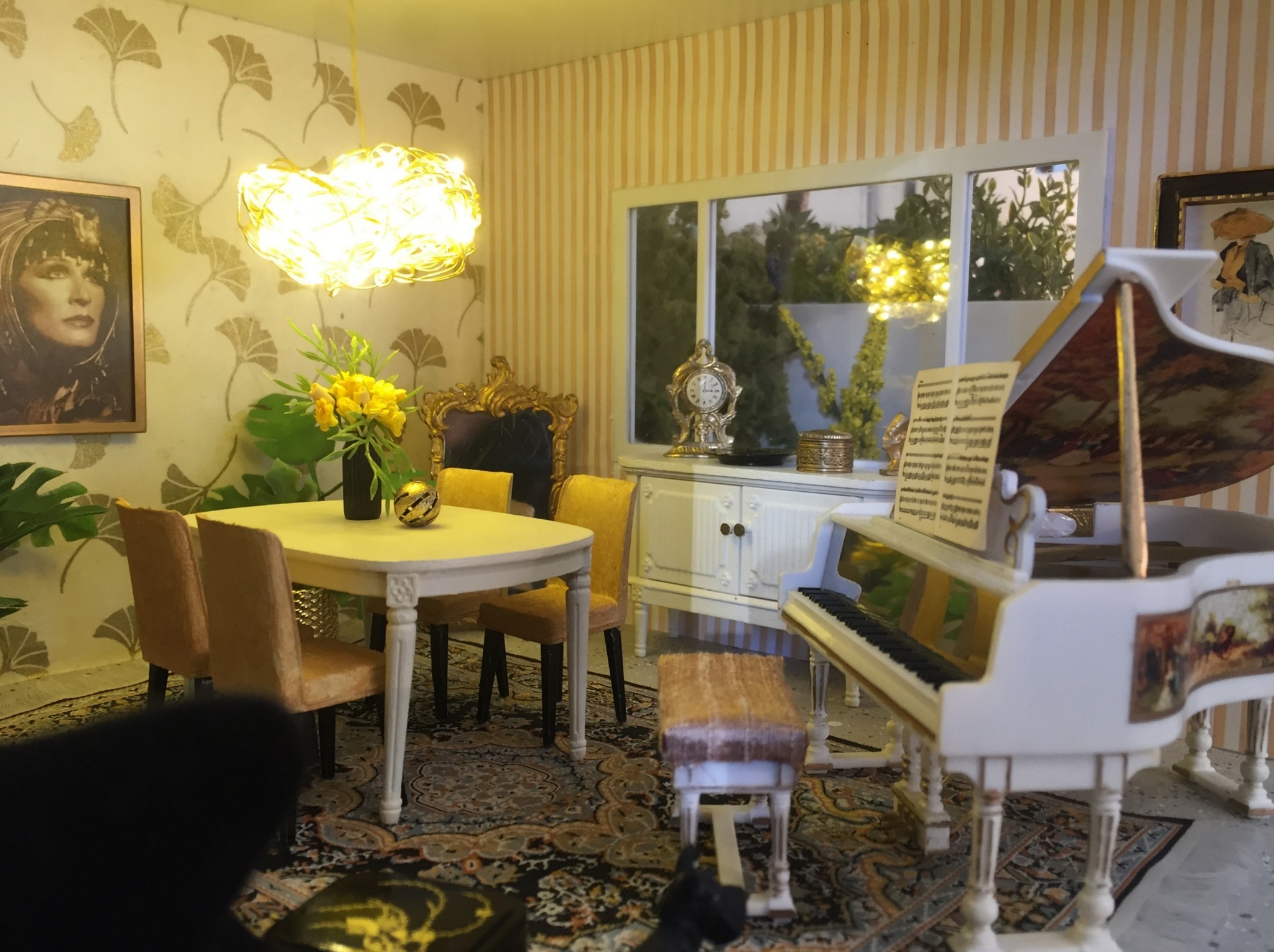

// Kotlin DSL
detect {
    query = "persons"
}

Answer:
[0,187,135,424]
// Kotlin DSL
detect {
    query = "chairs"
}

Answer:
[362,468,513,718]
[479,475,636,746]
[116,498,315,707]
[197,513,409,807]
[421,356,577,597]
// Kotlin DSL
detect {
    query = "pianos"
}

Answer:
[777,246,1274,952]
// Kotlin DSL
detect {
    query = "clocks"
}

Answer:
[663,338,743,458]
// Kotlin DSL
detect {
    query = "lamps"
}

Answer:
[236,0,481,296]
[837,178,979,328]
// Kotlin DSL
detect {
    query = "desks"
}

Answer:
[183,499,594,824]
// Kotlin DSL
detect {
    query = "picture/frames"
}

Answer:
[1154,164,1274,352]
[0,173,147,436]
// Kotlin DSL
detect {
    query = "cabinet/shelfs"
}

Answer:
[617,454,898,707]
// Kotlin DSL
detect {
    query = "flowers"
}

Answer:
[261,316,424,520]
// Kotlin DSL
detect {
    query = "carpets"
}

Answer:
[0,631,1198,952]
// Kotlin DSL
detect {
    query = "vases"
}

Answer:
[342,436,381,520]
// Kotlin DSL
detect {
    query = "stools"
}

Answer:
[657,651,805,917]
